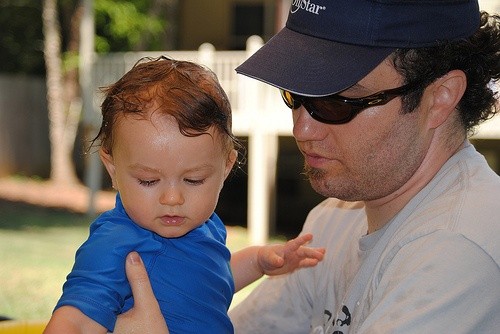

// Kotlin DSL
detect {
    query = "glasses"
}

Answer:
[279,82,417,126]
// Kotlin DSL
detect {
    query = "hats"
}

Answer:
[235,0,482,97]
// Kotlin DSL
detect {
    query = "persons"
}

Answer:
[108,0,500,333]
[42,56,326,334]
